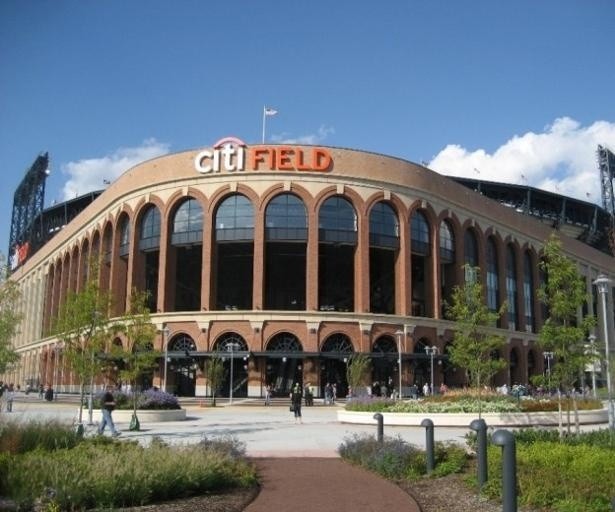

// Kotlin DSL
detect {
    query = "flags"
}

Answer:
[265,107,279,117]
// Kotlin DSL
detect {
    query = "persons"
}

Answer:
[370,374,448,400]
[0,381,53,413]
[498,383,547,398]
[263,385,274,407]
[98,384,122,438]
[290,383,341,423]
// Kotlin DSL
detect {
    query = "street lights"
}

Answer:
[225,343,240,403]
[163,326,170,392]
[588,334,597,401]
[591,275,615,428]
[542,351,554,393]
[424,345,438,396]
[394,330,404,400]
